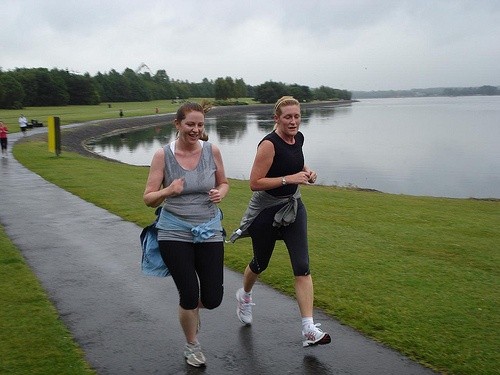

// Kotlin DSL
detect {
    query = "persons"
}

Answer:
[18,113,27,138]
[143,99,229,367]
[0,121,8,158]
[120,109,124,118]
[230,95,332,347]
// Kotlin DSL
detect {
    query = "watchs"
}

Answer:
[282,177,287,186]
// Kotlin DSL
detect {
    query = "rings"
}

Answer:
[313,178,316,181]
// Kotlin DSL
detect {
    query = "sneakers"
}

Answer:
[301,323,331,348]
[237,288,256,326]
[194,308,201,332]
[183,342,206,367]
